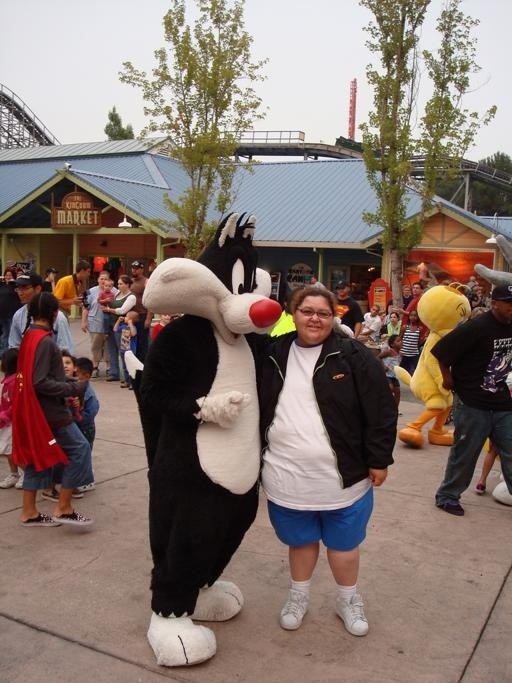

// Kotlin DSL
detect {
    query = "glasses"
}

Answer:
[296,306,333,319]
[14,287,32,292]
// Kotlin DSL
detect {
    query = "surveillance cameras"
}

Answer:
[65,163,72,171]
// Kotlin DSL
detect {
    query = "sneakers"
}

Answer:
[334,593,369,636]
[475,482,486,494]
[279,588,310,631]
[436,499,464,515]
[90,369,133,390]
[0,472,96,526]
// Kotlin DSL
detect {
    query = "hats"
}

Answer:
[131,260,144,268]
[492,285,512,301]
[14,271,43,285]
[334,279,346,288]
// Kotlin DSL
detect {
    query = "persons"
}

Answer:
[257,287,398,636]
[429,283,512,515]
[311,276,493,417]
[475,438,498,493]
[0,255,183,527]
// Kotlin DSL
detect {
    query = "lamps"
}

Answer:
[485,212,499,244]
[118,197,145,228]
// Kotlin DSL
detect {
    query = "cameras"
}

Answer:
[81,290,91,308]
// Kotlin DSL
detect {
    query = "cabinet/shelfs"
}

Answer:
[269,271,282,302]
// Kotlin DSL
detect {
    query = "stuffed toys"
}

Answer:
[124,212,283,666]
[394,285,472,448]
[473,235,512,507]
